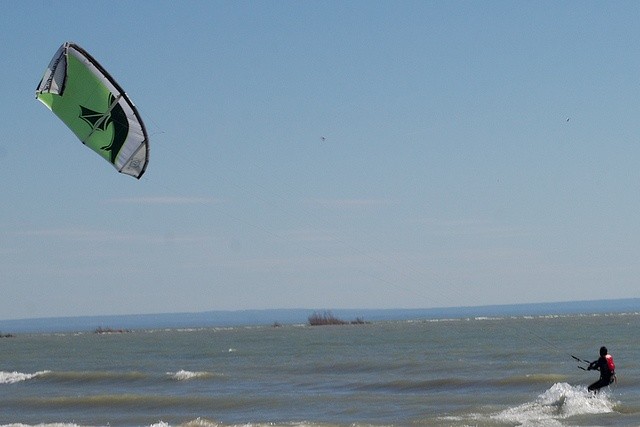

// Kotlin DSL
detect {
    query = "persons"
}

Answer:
[587,346,615,398]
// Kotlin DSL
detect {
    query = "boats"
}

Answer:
[309,312,348,324]
[94,325,133,333]
[351,316,371,323]
